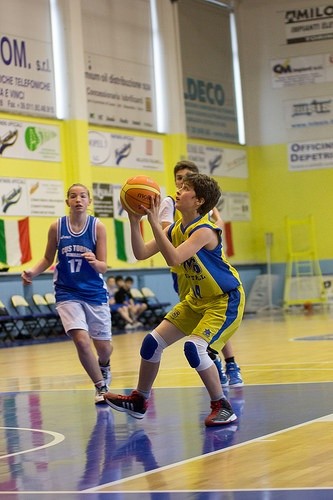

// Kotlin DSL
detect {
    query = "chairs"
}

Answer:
[0,287,172,345]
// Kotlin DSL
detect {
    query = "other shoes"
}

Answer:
[125,322,143,329]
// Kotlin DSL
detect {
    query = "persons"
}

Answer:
[105,274,147,331]
[20,184,113,404]
[104,171,245,425]
[154,160,244,387]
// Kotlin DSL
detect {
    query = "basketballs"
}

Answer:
[120,175,160,216]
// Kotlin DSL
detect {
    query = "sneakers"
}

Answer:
[213,357,227,387]
[225,362,243,387]
[205,395,238,426]
[94,380,109,405]
[102,391,149,420]
[98,358,112,386]
